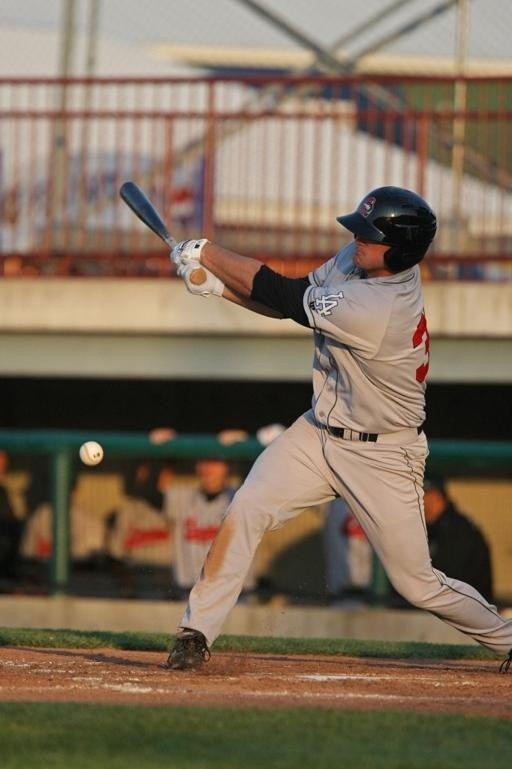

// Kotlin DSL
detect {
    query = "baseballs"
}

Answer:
[80,442,104,467]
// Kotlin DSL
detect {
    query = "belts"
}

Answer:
[328,427,378,442]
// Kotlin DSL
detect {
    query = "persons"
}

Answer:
[163,185,511,676]
[1,422,495,610]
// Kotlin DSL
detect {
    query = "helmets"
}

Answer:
[336,186,437,272]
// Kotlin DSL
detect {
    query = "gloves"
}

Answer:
[170,238,225,298]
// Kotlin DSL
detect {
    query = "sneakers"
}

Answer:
[167,630,210,671]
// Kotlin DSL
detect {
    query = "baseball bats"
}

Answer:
[118,181,207,286]
